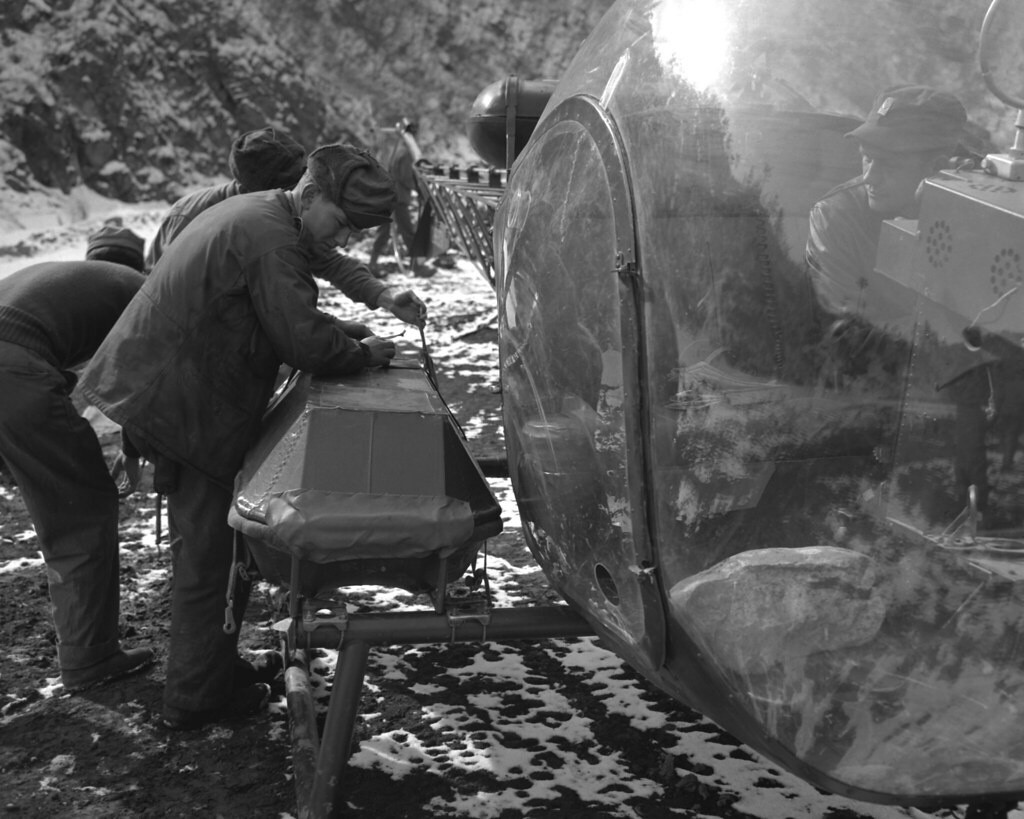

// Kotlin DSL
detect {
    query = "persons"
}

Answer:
[0,225,154,695]
[76,144,397,728]
[805,86,990,391]
[145,127,428,332]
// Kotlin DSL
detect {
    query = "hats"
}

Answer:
[843,84,967,149]
[228,127,309,192]
[87,227,145,261]
[307,144,399,229]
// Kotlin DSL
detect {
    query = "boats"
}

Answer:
[226,345,503,591]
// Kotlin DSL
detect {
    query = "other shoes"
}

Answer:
[225,652,283,688]
[63,645,156,692]
[165,682,272,729]
